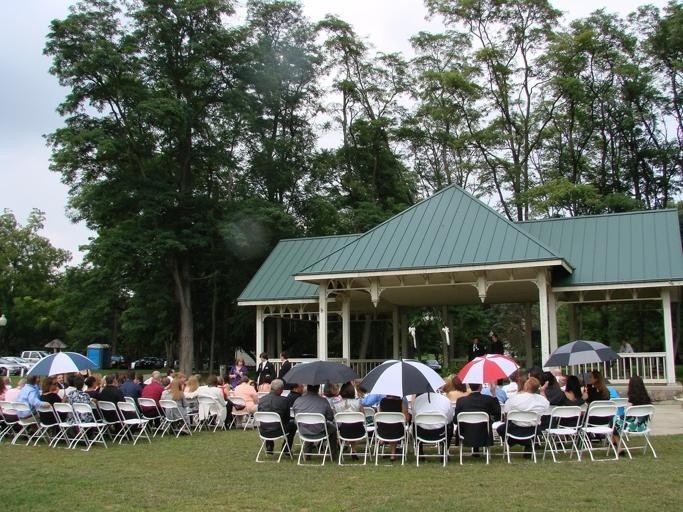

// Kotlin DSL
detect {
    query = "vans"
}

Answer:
[21,350,50,364]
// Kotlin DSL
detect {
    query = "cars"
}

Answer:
[0,357,26,376]
[5,355,35,371]
[421,358,442,372]
[109,353,208,371]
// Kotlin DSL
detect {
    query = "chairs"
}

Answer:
[87,391,271,447]
[0,400,109,452]
[605,404,658,461]
[331,410,373,466]
[289,396,633,455]
[373,411,410,467]
[541,405,583,465]
[570,405,621,463]
[413,411,449,468]
[497,409,540,465]
[254,411,294,464]
[456,410,492,467]
[294,412,333,467]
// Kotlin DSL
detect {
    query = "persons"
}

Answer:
[1,352,292,446]
[468,335,504,362]
[258,370,652,461]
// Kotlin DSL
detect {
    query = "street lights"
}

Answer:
[0,313,8,332]
